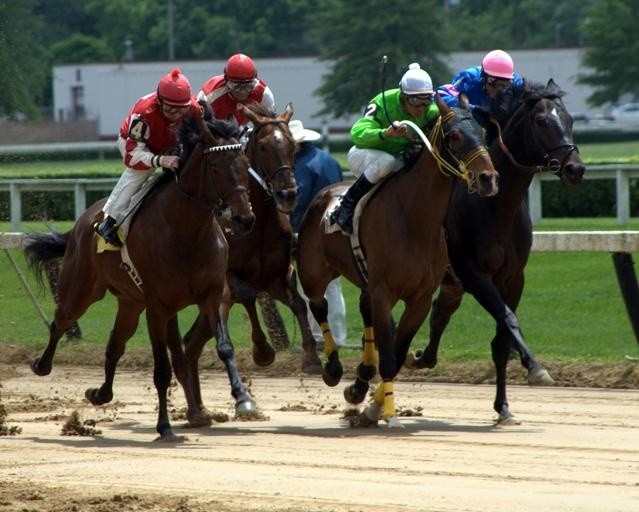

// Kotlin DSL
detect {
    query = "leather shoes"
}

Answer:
[97,216,123,248]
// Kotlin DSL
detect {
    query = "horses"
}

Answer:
[358,76,588,425]
[164,102,324,428]
[22,113,259,444]
[296,89,503,430]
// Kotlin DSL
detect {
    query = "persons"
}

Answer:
[434,49,525,147]
[196,53,276,152]
[288,119,346,351]
[330,63,441,237]
[94,70,203,247]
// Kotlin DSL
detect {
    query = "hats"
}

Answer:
[157,68,193,107]
[287,120,321,141]
[398,62,434,96]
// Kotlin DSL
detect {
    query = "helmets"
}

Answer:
[224,53,257,83]
[482,49,515,81]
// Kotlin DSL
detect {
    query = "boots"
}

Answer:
[331,171,375,235]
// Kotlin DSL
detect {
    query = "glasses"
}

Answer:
[399,82,432,107]
[161,102,192,116]
[484,74,512,89]
[227,80,257,93]
